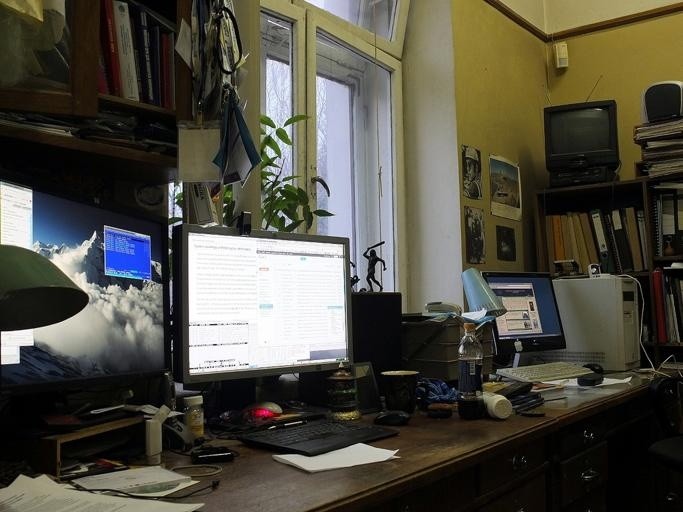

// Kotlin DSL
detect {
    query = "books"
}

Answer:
[539,114,682,347]
[529,382,567,402]
[0,1,184,159]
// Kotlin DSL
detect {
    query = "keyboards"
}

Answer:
[495,361,595,383]
[235,419,401,457]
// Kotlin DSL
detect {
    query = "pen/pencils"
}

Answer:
[268,419,308,430]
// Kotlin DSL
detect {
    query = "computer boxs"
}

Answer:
[542,275,641,372]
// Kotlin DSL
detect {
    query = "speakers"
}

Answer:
[588,263,602,278]
[643,81,683,123]
[553,43,568,69]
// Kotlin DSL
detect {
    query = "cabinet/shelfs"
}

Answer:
[0,1,195,174]
[529,165,682,370]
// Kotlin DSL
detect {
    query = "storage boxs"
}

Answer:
[402,312,493,384]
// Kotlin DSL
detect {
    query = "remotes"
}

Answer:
[494,382,533,397]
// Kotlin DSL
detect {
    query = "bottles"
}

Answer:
[457,323,485,418]
[181,395,204,440]
[480,391,512,420]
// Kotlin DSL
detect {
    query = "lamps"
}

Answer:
[457,266,508,364]
[0,243,91,334]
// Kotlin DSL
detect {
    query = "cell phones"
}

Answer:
[577,373,603,385]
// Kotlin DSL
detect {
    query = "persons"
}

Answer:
[463,208,485,264]
[461,146,483,200]
[362,247,387,291]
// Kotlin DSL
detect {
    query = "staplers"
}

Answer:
[425,301,463,316]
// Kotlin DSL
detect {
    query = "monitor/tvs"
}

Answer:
[0,180,172,429]
[544,99,619,171]
[480,271,567,367]
[171,224,354,427]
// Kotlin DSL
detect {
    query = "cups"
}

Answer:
[381,370,428,414]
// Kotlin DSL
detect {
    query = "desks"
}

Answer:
[0,362,682,512]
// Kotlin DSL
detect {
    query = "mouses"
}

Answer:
[374,410,412,427]
[582,363,604,374]
[242,401,283,417]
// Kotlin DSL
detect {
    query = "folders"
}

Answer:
[588,209,617,274]
